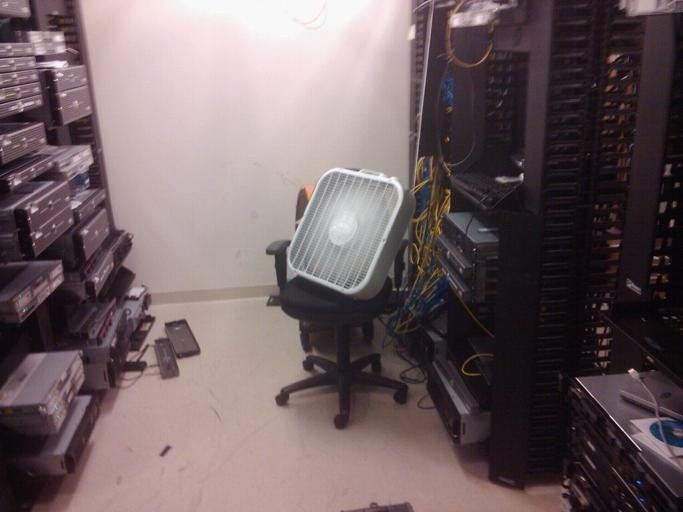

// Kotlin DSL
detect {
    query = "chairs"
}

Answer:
[264,182,408,429]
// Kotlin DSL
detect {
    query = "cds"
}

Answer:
[648,419,683,448]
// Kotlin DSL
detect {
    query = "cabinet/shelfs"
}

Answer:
[0,0,116,512]
